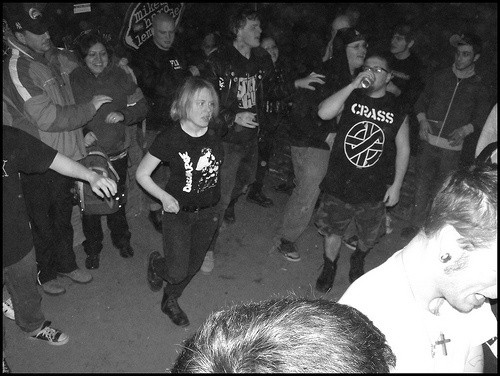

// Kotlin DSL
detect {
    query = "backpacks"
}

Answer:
[75,146,127,216]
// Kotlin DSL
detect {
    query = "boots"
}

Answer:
[313,253,340,301]
[348,243,373,283]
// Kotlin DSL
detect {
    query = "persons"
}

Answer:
[3,4,212,319]
[171,295,396,373]
[315,52,412,292]
[136,79,225,327]
[3,125,117,346]
[381,25,488,238]
[335,167,497,374]
[192,9,367,271]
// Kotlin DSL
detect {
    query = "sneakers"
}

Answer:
[24,320,70,346]
[147,251,163,292]
[3,296,15,320]
[41,280,66,295]
[161,293,189,326]
[3,357,12,373]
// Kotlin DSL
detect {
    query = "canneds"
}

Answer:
[360,77,376,88]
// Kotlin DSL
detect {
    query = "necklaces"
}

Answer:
[428,327,450,358]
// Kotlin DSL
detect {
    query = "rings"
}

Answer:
[113,117,115,119]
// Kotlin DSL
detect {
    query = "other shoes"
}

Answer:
[385,225,393,235]
[272,234,302,262]
[115,243,134,258]
[247,184,273,208]
[148,208,163,233]
[224,208,235,223]
[56,267,94,284]
[200,251,215,272]
[343,235,359,250]
[85,254,99,269]
[316,228,328,239]
[272,184,295,193]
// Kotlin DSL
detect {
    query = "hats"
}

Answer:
[344,28,372,44]
[449,33,482,51]
[8,8,55,35]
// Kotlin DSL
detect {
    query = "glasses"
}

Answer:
[363,65,389,74]
